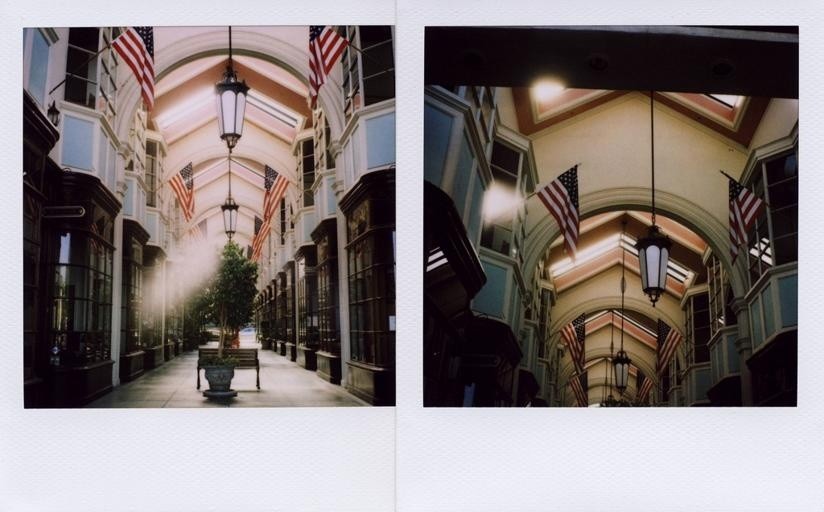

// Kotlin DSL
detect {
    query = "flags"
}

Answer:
[167,162,195,224]
[189,218,207,246]
[308,25,349,110]
[728,179,764,266]
[656,318,684,377]
[569,371,588,407]
[110,26,154,117]
[559,312,586,375]
[637,369,654,407]
[536,164,580,264]
[263,164,289,224]
[250,215,271,262]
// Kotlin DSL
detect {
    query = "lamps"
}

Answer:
[220,155,238,240]
[611,249,632,394]
[632,91,674,310]
[211,27,252,155]
[47,99,60,128]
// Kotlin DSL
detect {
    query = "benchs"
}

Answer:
[194,348,259,392]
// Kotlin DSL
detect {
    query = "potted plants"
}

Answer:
[202,354,237,402]
